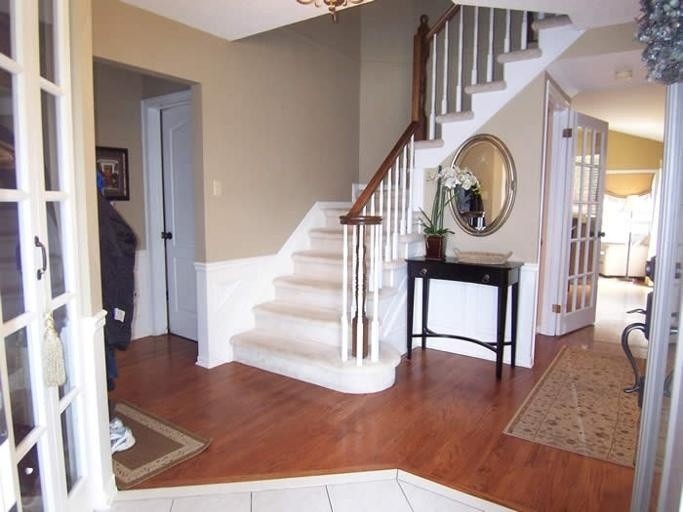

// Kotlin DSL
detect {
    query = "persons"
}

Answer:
[0,127,67,488]
[97,169,136,457]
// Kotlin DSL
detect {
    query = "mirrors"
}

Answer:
[447,134,517,237]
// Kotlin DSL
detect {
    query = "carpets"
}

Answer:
[502,344,647,467]
[109,400,214,491]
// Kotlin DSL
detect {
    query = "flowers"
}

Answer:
[453,165,483,214]
[415,164,463,236]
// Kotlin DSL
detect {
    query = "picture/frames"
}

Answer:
[96,147,129,200]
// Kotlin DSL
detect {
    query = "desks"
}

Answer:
[404,256,524,377]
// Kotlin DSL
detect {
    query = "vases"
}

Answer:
[425,237,447,261]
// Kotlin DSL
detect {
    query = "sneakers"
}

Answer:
[109,416,136,455]
